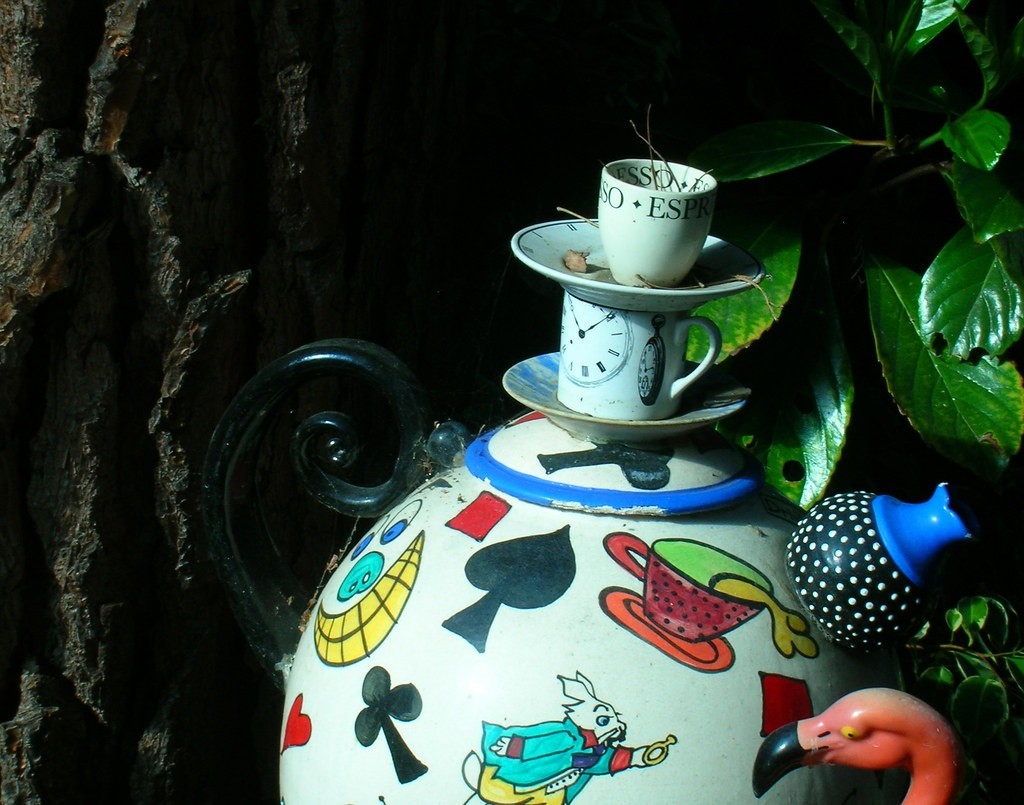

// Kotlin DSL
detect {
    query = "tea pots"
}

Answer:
[190,336,984,805]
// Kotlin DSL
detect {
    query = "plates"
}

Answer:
[501,350,752,443]
[508,219,766,313]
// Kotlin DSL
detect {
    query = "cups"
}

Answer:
[596,158,718,290]
[557,289,722,421]
